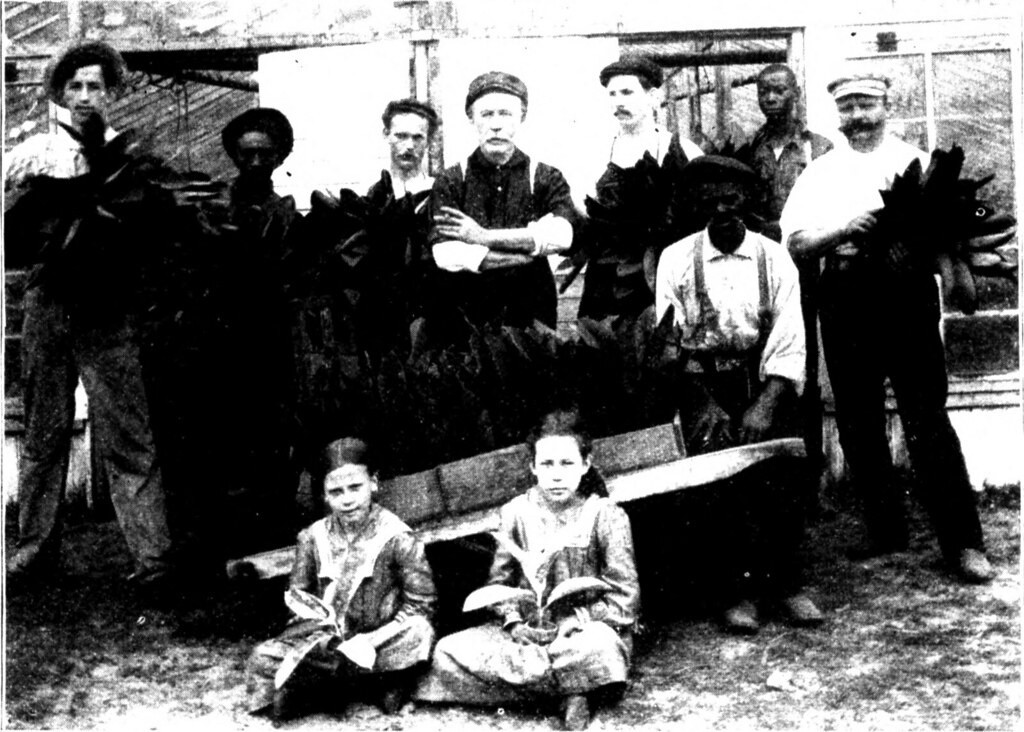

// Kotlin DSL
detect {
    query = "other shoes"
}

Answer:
[725,600,759,631]
[783,595,823,624]
[953,548,991,580]
[560,694,590,730]
[845,526,904,556]
[378,686,401,714]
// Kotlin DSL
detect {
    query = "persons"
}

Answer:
[243,435,437,722]
[572,57,996,635]
[6,39,574,611]
[433,407,643,731]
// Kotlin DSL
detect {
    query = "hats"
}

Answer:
[680,156,758,200]
[600,58,662,88]
[382,100,437,128]
[44,36,129,107]
[465,71,529,112]
[222,107,293,164]
[827,71,892,100]
